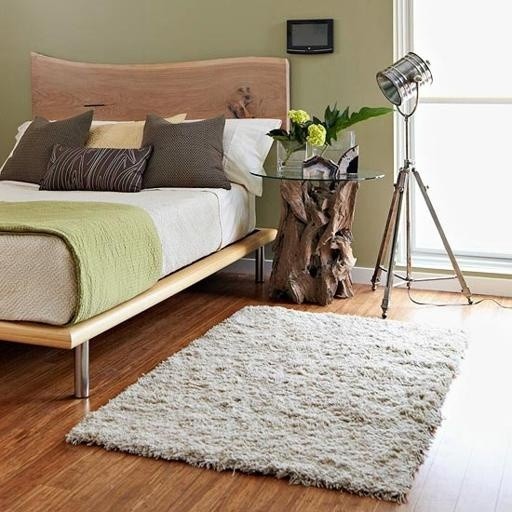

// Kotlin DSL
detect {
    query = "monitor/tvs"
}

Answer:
[287,19,334,53]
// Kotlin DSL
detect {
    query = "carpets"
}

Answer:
[65,304,467,504]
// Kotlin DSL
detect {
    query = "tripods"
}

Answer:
[372,160,473,317]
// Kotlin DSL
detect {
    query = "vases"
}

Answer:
[276,130,355,173]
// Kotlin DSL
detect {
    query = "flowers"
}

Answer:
[263,103,397,164]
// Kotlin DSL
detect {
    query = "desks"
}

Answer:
[247,168,385,304]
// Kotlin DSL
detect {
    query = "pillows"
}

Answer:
[0,110,282,197]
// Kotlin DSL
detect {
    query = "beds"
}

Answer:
[0,51,291,399]
